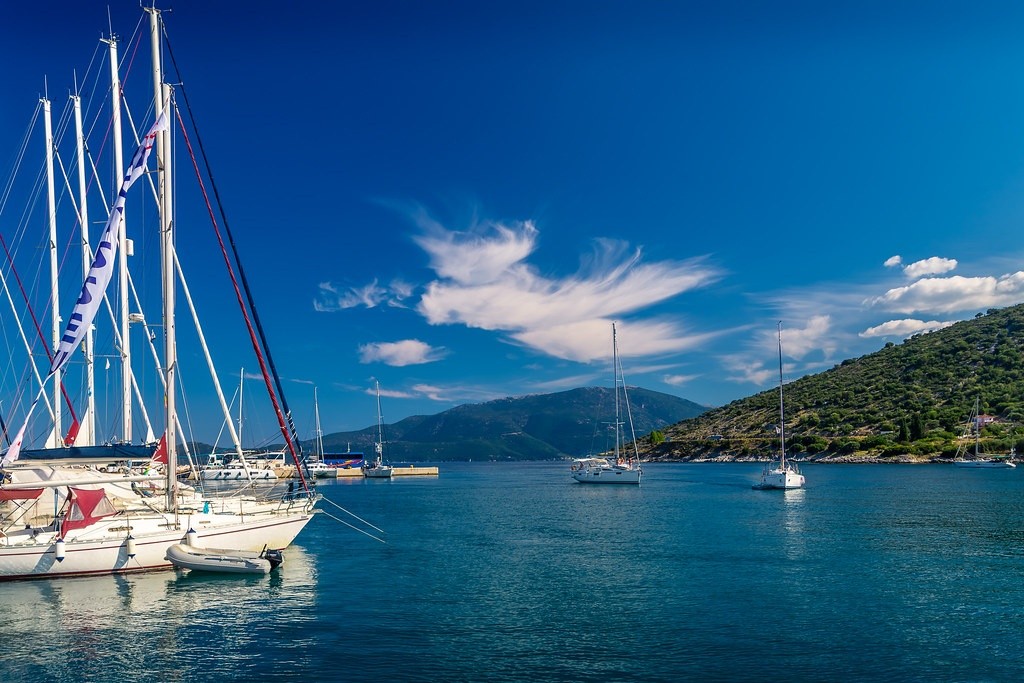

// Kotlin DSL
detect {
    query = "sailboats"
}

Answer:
[0,0,439,581]
[950,396,1016,469]
[568,320,642,486]
[752,319,806,489]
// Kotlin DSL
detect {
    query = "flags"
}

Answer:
[4,420,79,465]
[143,432,168,475]
